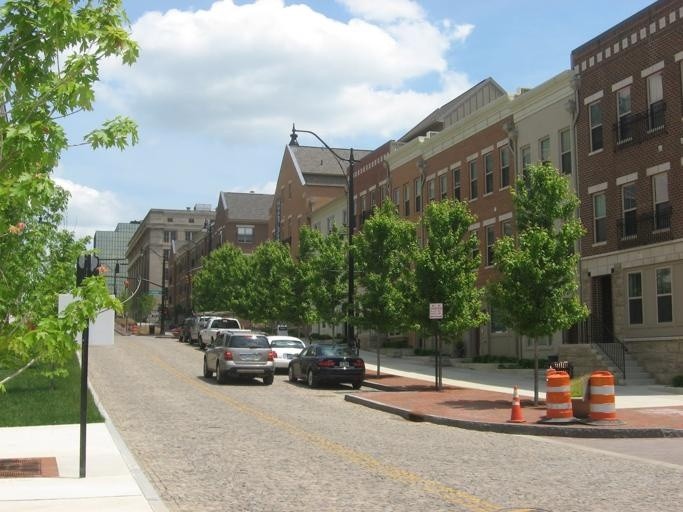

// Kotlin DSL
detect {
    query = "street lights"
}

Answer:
[289,123,360,347]
[202,218,212,258]
[140,246,166,335]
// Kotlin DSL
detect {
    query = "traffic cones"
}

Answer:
[506,386,526,423]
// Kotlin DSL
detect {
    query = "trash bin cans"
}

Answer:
[149,325,154,334]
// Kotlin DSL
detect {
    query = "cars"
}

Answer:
[179,316,365,390]
